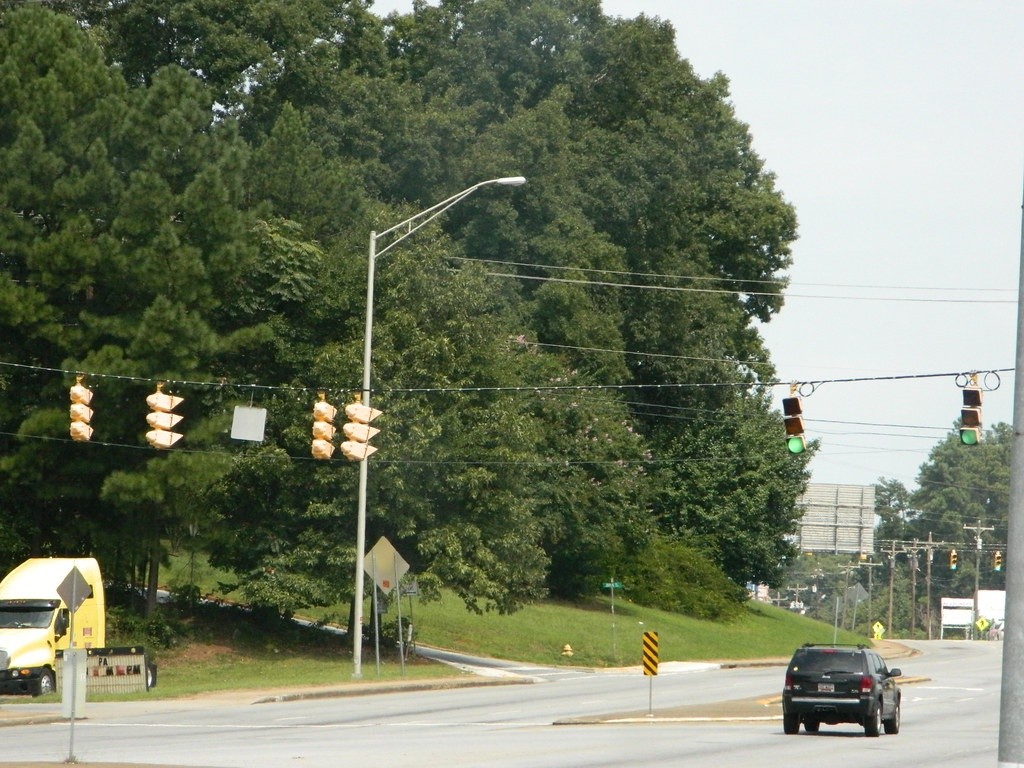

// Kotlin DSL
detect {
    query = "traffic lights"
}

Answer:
[950,549,957,570]
[994,550,1002,570]
[340,402,384,460]
[144,392,185,450]
[782,395,806,456]
[960,385,983,446]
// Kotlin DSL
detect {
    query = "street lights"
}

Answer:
[353,174,526,678]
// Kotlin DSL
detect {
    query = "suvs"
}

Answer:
[782,644,903,737]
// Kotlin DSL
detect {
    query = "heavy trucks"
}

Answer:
[0,557,157,698]
